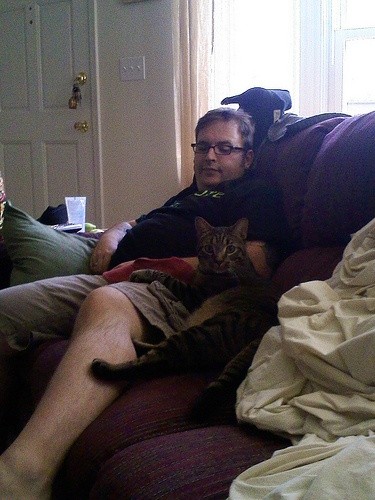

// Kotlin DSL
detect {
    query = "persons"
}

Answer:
[0,107,299,496]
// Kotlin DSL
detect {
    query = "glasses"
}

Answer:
[190,142,246,157]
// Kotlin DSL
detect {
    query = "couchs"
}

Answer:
[21,112,375,499]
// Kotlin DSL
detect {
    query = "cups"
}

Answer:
[65,196,86,233]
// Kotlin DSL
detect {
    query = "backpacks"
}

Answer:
[220,87,292,153]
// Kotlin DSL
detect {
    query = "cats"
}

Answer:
[92,217,275,423]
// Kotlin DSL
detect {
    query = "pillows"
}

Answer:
[1,200,96,289]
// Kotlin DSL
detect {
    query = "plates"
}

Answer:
[62,228,82,233]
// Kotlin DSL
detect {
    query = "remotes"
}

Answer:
[52,222,83,233]
